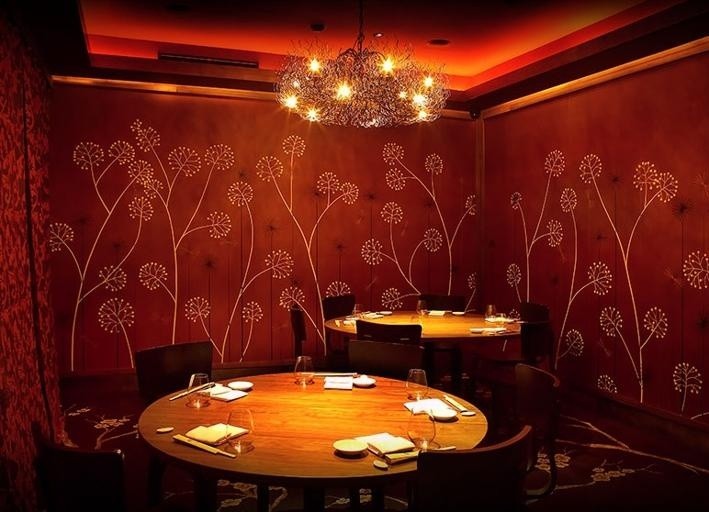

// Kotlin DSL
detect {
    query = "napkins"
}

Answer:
[402,397,452,414]
[355,431,414,458]
[187,417,248,448]
[323,376,354,390]
[198,385,248,403]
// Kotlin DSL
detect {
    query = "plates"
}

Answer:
[333,439,368,457]
[452,311,466,316]
[228,381,253,392]
[353,377,376,388]
[380,311,392,315]
[430,408,457,422]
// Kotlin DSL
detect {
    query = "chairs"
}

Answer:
[134,340,211,472]
[32,421,129,509]
[322,293,357,358]
[486,323,554,409]
[289,303,335,367]
[352,317,421,367]
[420,295,466,384]
[403,427,533,511]
[474,300,555,384]
[486,360,558,483]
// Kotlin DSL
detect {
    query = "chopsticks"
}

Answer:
[169,382,216,401]
[386,446,457,466]
[173,434,236,459]
[298,371,357,378]
[443,394,468,412]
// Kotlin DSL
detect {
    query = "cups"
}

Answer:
[352,304,362,322]
[188,373,210,408]
[295,356,313,385]
[487,304,496,322]
[408,408,434,455]
[417,300,426,316]
[405,369,428,401]
[225,407,253,453]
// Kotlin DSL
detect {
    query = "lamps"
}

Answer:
[272,0,451,129]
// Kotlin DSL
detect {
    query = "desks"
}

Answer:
[324,309,525,376]
[140,371,488,506]
